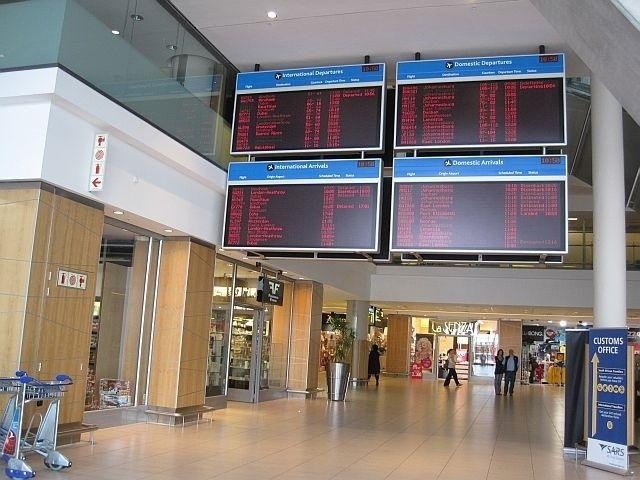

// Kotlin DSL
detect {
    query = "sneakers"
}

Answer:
[444,382,464,387]
[495,391,515,397]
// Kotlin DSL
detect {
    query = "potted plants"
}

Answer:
[326,316,358,402]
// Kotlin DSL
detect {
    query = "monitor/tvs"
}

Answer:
[369,306,376,326]
[322,313,346,331]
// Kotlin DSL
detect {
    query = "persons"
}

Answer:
[529,353,539,384]
[368,344,382,386]
[501,349,519,395]
[495,349,503,395]
[443,349,463,386]
[415,337,432,358]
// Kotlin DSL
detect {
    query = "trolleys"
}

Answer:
[0,369,74,480]
